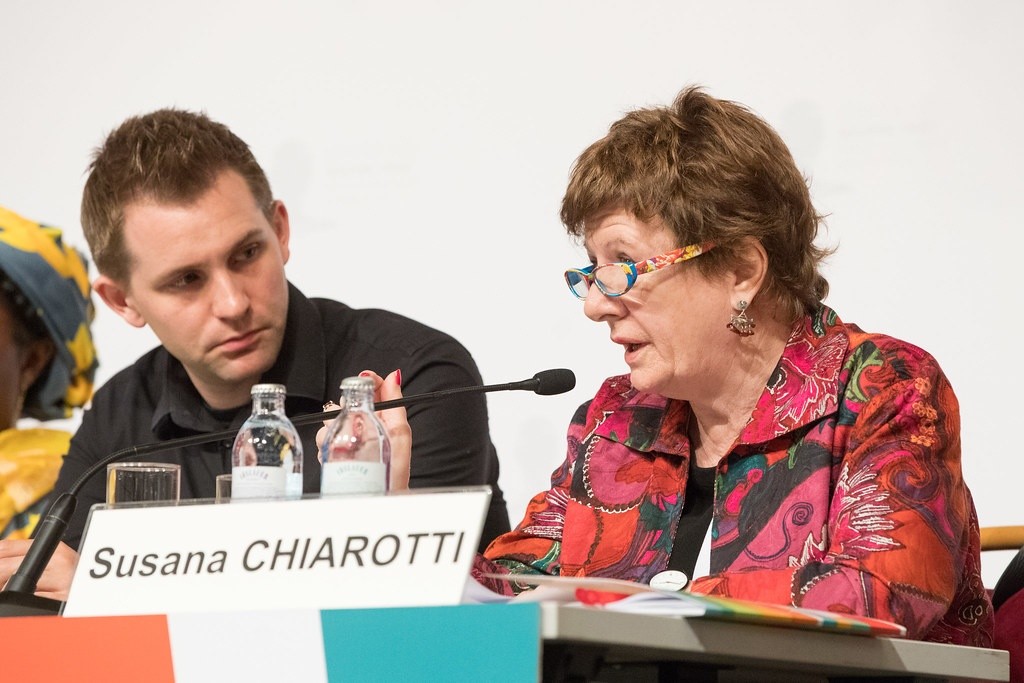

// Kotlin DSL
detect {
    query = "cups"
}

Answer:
[106,462,181,506]
[215,473,231,503]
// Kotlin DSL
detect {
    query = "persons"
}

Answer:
[0,109,513,602]
[315,87,992,648]
[0,206,100,539]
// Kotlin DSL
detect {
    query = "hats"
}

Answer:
[0,206,100,423]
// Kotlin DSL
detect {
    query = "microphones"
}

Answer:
[0,369,577,617]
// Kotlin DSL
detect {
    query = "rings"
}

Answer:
[323,400,336,411]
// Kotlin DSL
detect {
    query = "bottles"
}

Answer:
[320,376,392,495]
[230,384,304,498]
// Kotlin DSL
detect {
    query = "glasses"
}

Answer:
[558,239,731,298]
